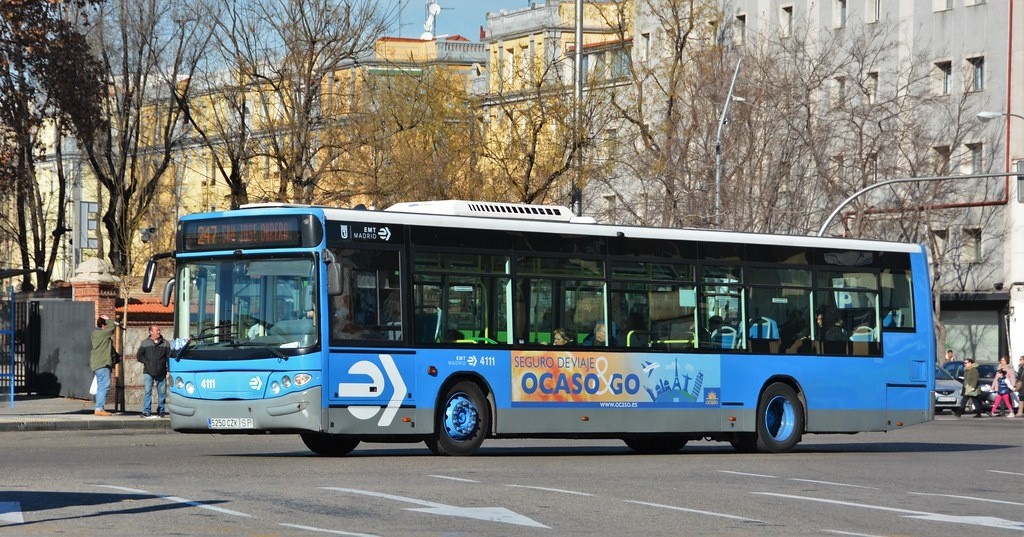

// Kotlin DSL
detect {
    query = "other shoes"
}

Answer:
[138,414,150,418]
[985,411,993,416]
[1016,412,1024,416]
[1007,413,1014,417]
[996,412,1005,416]
[158,415,168,418]
[95,411,112,416]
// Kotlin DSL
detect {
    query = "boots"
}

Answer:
[973,410,981,417]
[950,407,964,418]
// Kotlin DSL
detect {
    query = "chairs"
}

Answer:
[380,304,902,356]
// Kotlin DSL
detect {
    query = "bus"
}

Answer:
[141,197,939,460]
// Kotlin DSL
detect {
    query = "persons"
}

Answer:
[302,294,316,326]
[1013,355,1024,417]
[811,308,838,343]
[540,326,575,346]
[990,355,1018,419]
[88,314,121,415]
[137,324,171,418]
[954,358,983,418]
[944,349,956,362]
[985,369,1015,418]
[583,319,607,347]
[775,308,805,337]
[706,300,739,336]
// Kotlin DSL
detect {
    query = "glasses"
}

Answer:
[964,362,970,364]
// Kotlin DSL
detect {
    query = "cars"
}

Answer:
[934,358,1023,415]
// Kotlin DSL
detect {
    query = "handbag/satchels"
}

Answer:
[964,385,979,396]
[89,375,98,395]
[1010,392,1021,408]
[112,347,120,365]
